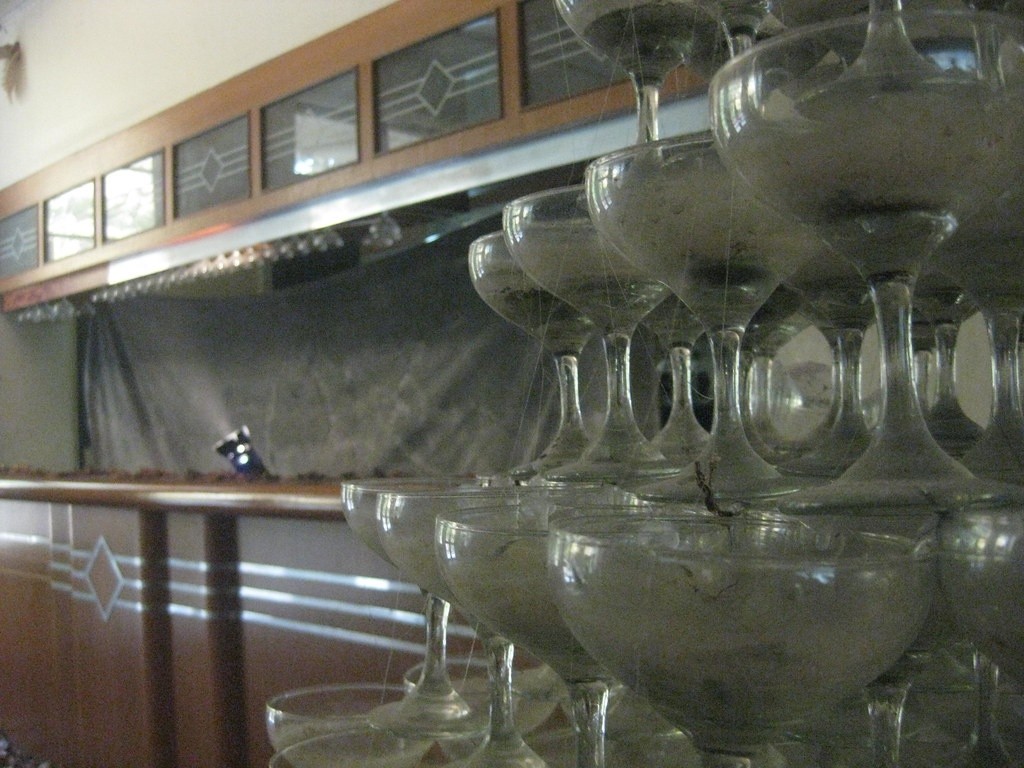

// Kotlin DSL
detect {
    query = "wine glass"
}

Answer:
[263,0,1024,768]
